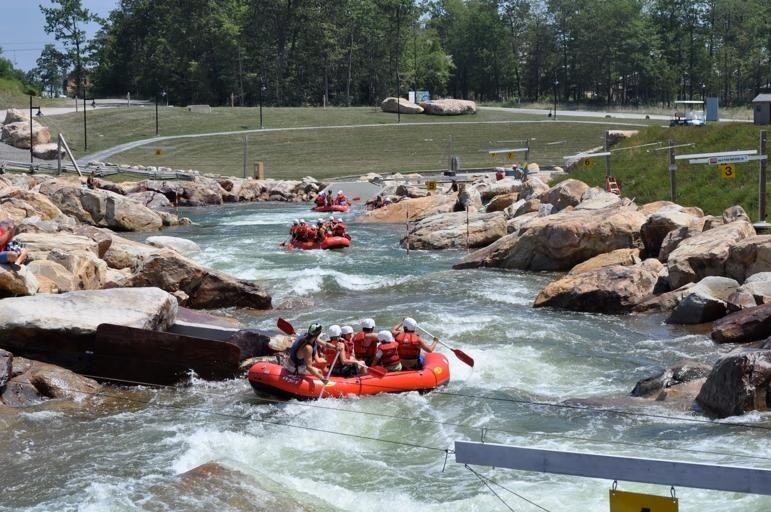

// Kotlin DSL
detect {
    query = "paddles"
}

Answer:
[278,318,298,336]
[351,356,386,378]
[280,234,291,247]
[349,197,361,202]
[417,325,475,366]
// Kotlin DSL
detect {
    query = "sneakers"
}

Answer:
[11,262,20,271]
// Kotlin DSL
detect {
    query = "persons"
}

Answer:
[370,330,402,375]
[342,325,356,359]
[284,321,329,385]
[313,189,351,208]
[0,223,29,270]
[319,325,366,376]
[392,316,439,370]
[289,214,348,242]
[352,317,378,366]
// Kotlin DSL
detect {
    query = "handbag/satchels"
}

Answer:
[7,240,21,256]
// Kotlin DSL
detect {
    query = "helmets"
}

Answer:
[307,322,321,336]
[499,168,504,172]
[402,318,416,332]
[318,189,343,196]
[513,164,517,168]
[362,318,375,329]
[328,325,340,337]
[292,216,342,230]
[340,326,353,335]
[377,330,392,343]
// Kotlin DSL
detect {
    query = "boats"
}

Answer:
[289,236,349,249]
[247,352,450,402]
[313,204,349,212]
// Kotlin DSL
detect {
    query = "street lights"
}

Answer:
[258,73,265,131]
[82,96,98,149]
[394,72,402,122]
[552,72,560,118]
[154,86,166,135]
[27,93,43,161]
